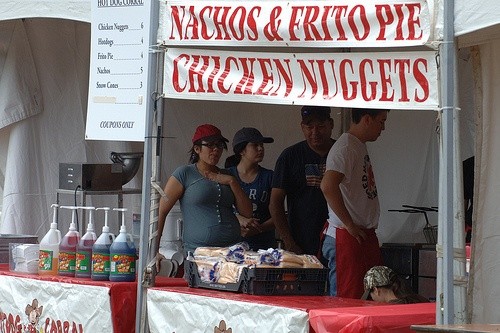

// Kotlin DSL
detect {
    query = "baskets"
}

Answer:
[423,225,437,243]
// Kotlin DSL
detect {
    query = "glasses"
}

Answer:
[203,141,224,149]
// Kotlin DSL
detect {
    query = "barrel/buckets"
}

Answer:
[159,201,184,259]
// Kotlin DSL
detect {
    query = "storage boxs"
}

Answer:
[185,259,328,297]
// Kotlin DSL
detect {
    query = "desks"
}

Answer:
[0,262,190,333]
[149,286,437,333]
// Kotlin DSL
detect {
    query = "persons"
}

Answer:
[320,109,390,300]
[461,156,476,243]
[154,105,338,274]
[363,266,432,304]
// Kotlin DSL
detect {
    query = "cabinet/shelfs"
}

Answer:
[53,189,142,236]
[380,242,437,300]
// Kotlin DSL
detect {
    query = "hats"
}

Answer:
[361,265,397,300]
[301,106,331,122]
[233,128,274,148]
[192,125,230,143]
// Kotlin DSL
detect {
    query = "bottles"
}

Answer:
[58,223,80,276]
[91,226,115,280]
[38,223,61,276]
[74,224,97,277]
[109,226,137,281]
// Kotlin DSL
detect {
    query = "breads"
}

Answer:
[193,247,323,268]
